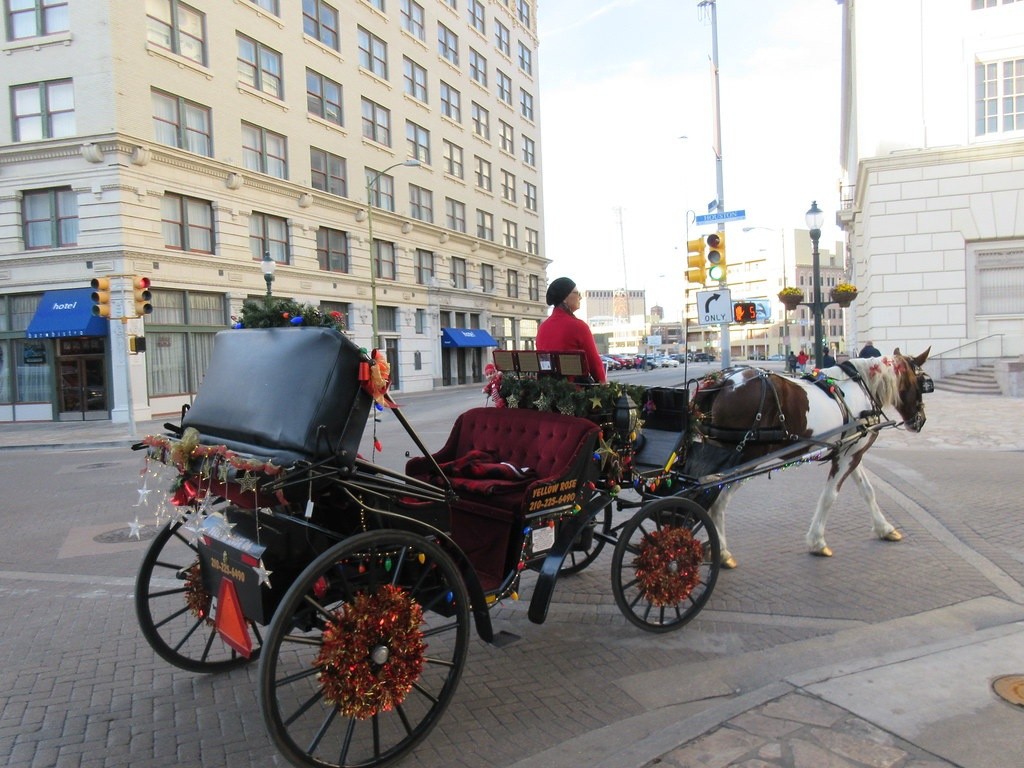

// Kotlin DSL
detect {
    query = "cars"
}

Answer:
[59,370,107,410]
[598,353,716,370]
[747,352,766,360]
[769,354,786,361]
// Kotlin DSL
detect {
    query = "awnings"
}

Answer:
[25,287,109,338]
[441,327,498,348]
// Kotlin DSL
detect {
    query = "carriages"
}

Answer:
[133,300,935,768]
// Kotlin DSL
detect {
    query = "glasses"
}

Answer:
[570,291,580,295]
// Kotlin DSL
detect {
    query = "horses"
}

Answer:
[686,346,934,567]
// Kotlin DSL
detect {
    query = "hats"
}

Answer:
[823,347,829,353]
[547,277,576,305]
[866,340,873,346]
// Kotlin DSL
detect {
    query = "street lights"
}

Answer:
[261,250,277,302]
[777,202,859,370]
[364,158,423,349]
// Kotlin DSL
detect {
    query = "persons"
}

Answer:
[823,347,836,369]
[635,357,645,372]
[859,340,881,358]
[788,351,808,378]
[536,277,606,392]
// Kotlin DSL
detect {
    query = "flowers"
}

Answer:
[778,287,803,297]
[831,283,857,294]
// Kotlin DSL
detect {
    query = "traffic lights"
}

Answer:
[733,302,760,324]
[130,336,146,353]
[133,276,154,316]
[706,230,728,284]
[685,236,706,286]
[90,276,111,320]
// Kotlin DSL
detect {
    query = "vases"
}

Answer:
[831,293,858,307]
[779,296,804,310]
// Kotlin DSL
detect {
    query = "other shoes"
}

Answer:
[632,433,645,455]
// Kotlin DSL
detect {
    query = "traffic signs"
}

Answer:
[694,289,733,325]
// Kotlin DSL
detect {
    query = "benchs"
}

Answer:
[405,407,600,523]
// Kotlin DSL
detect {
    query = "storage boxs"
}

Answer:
[197,505,330,626]
